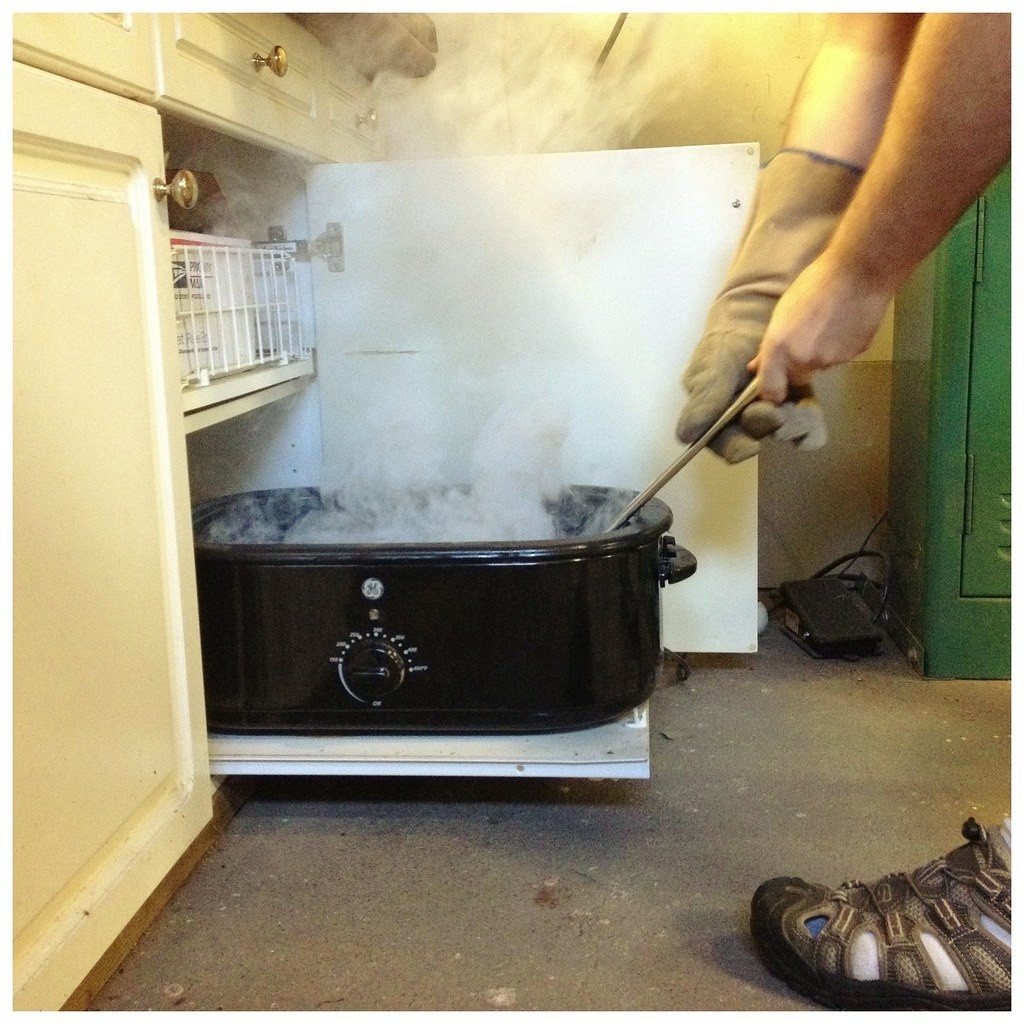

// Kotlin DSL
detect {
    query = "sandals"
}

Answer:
[755,820,1013,1010]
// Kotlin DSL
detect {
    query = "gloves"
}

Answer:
[677,149,868,463]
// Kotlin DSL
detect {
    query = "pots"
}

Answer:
[192,484,698,736]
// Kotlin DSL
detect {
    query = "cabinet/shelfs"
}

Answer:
[13,14,768,1006]
[877,157,1010,682]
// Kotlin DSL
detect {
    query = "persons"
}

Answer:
[677,12,1011,1012]
